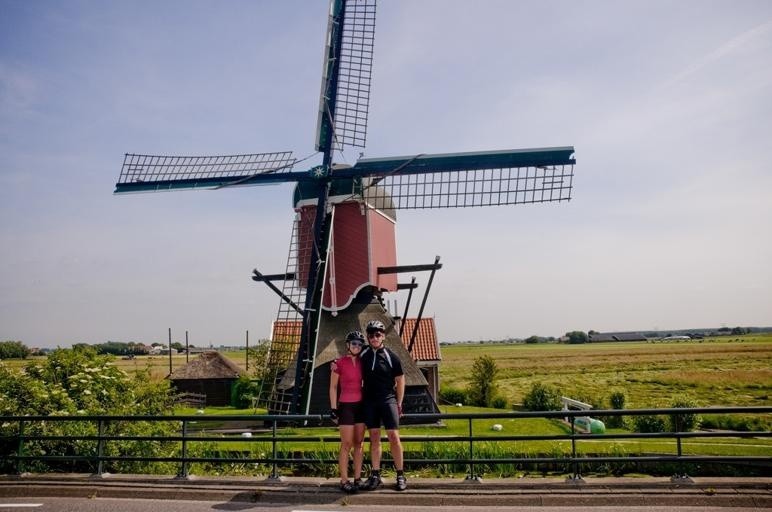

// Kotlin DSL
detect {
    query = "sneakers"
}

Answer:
[340,473,381,494]
[397,476,407,490]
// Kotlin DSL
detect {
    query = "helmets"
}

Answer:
[345,331,365,343]
[366,321,385,333]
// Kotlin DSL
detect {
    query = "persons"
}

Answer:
[329,320,408,494]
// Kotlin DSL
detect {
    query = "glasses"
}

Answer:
[352,343,362,346]
[369,333,383,338]
